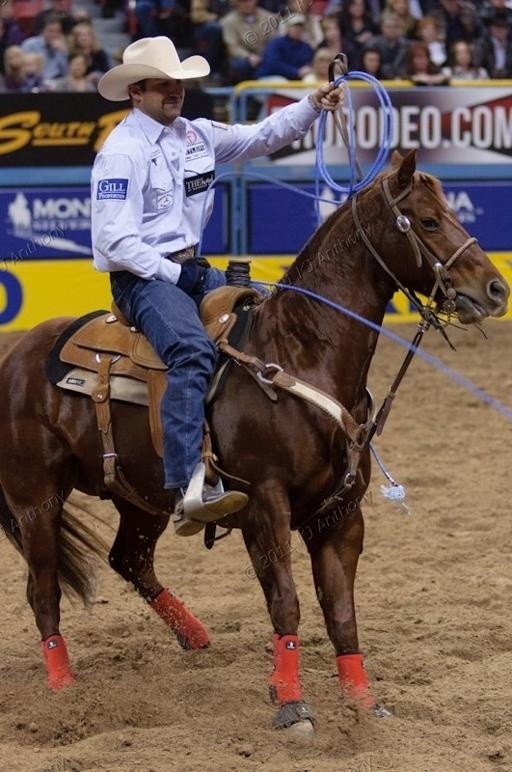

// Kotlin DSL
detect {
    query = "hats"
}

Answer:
[97,36,211,102]
[287,13,306,25]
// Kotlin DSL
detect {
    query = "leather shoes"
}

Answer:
[173,484,249,536]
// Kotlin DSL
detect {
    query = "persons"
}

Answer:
[0,0,512,94]
[91,34,343,536]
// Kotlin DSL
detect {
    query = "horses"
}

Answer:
[0,147,511,751]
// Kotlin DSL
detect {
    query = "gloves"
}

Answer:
[175,257,211,296]
